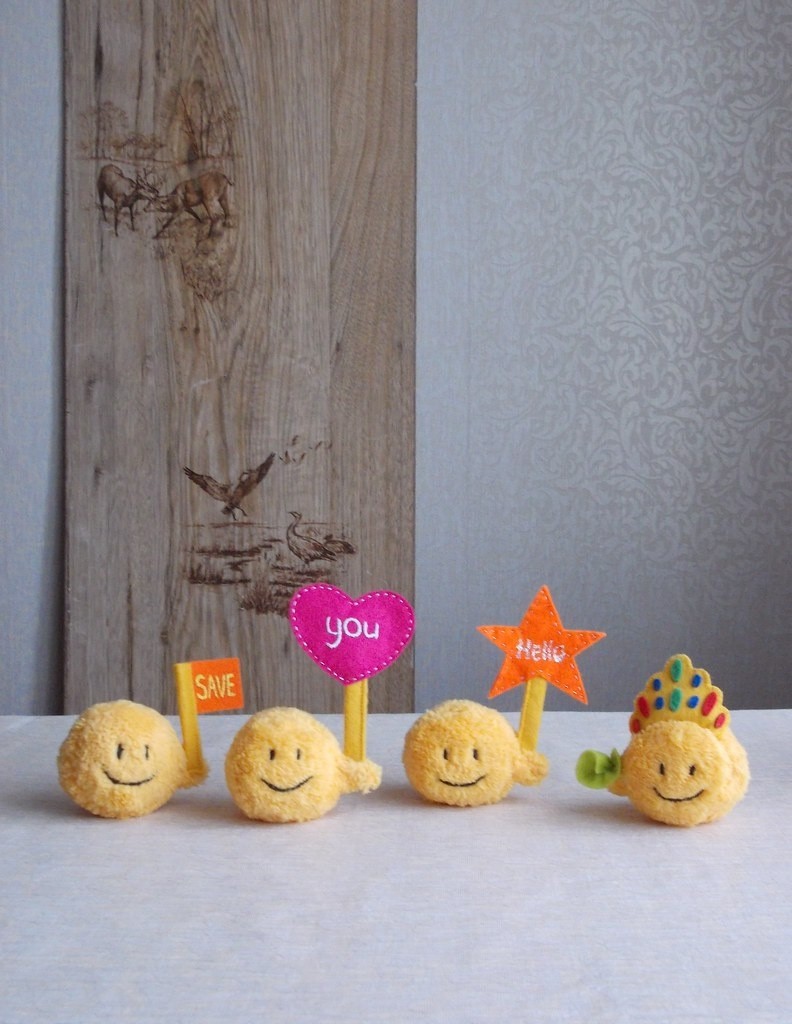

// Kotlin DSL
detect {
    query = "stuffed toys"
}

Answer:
[225,704,383,823]
[575,653,750,828]
[57,700,209,818]
[402,696,549,808]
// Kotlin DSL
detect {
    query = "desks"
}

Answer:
[0,704,792,1024]
